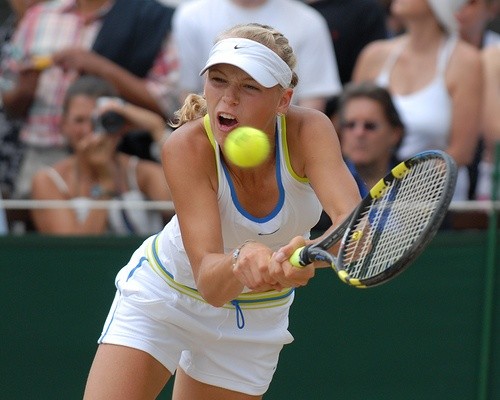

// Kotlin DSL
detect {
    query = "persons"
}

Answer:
[452,1,499,48]
[172,1,344,119]
[0,0,177,234]
[0,0,38,235]
[353,0,483,202]
[477,45,500,202]
[81,17,375,399]
[311,81,407,230]
[30,74,177,235]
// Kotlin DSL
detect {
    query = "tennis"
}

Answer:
[224,126,270,168]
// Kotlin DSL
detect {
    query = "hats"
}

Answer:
[428,0,467,37]
[199,37,294,91]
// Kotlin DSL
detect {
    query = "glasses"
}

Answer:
[339,120,380,130]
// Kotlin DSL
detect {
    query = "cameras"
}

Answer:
[91,112,125,135]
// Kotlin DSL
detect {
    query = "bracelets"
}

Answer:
[231,239,252,266]
[90,186,118,201]
